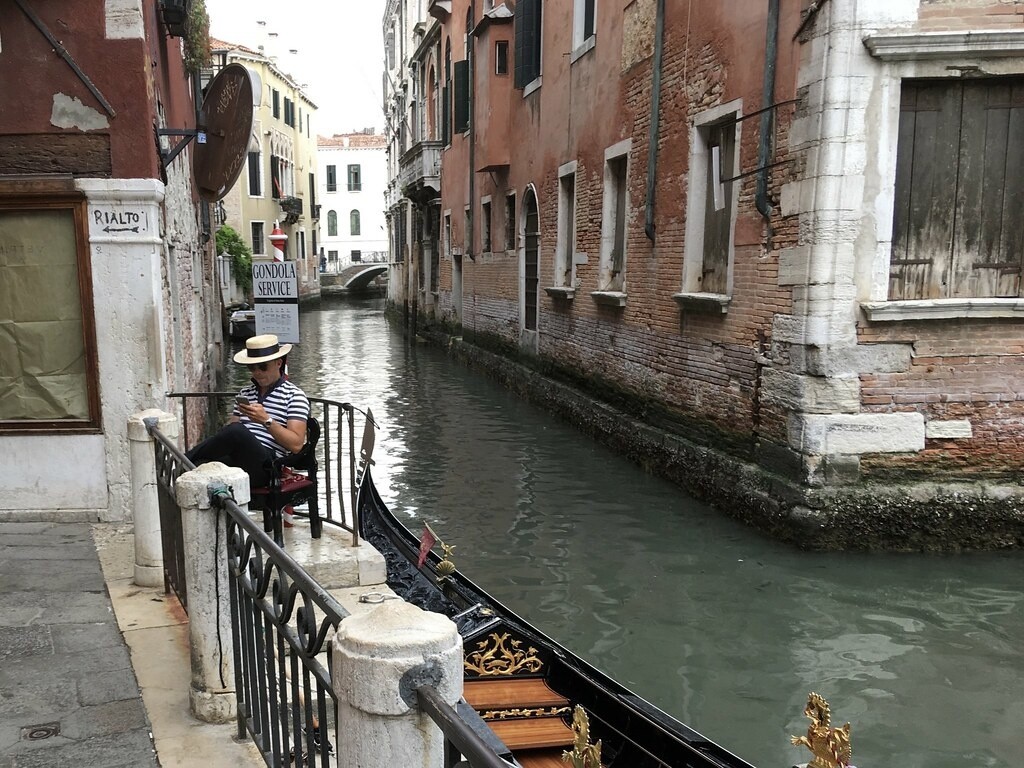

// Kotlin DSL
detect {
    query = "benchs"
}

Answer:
[461,677,579,751]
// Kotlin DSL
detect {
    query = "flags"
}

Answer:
[417,527,437,569]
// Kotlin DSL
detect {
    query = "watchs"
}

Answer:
[264,417,273,428]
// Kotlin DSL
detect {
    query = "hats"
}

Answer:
[233,333,293,364]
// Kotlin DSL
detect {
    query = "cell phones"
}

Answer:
[236,396,250,406]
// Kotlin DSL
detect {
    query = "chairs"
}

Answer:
[250,418,323,549]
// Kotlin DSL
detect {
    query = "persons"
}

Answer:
[181,334,310,489]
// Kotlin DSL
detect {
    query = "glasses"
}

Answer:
[246,363,268,372]
